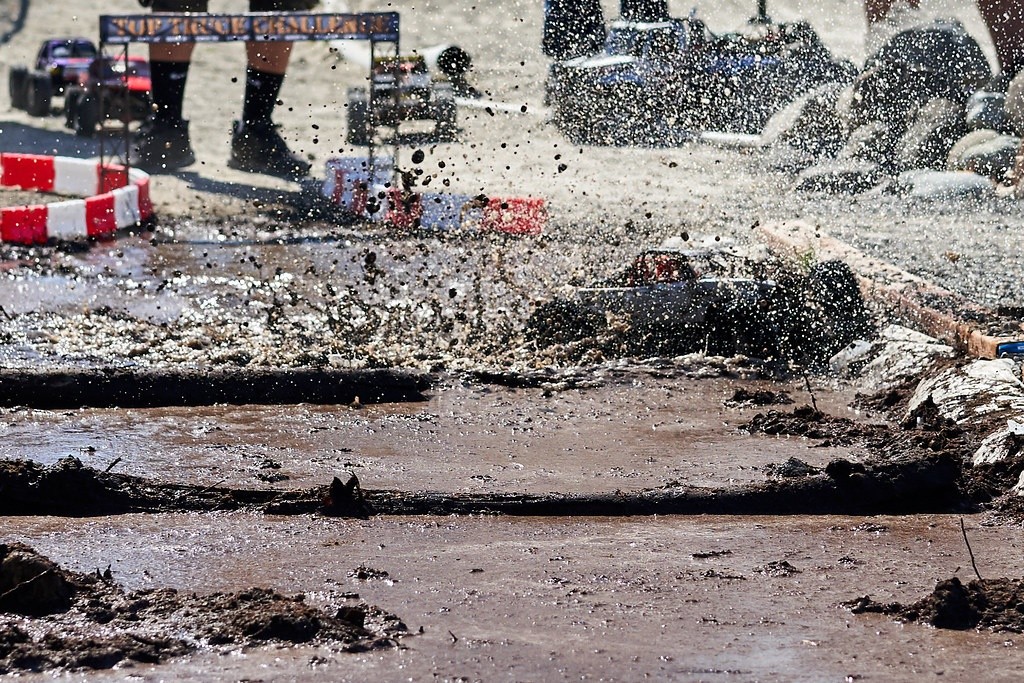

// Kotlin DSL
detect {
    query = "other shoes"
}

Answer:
[110,119,196,174]
[227,120,310,182]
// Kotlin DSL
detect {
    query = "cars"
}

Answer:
[549,19,785,122]
[529,240,798,350]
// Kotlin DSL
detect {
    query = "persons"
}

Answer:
[542,0,669,105]
[119,0,319,181]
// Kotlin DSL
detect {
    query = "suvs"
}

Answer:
[7,38,98,120]
[345,50,460,149]
[66,55,158,139]
